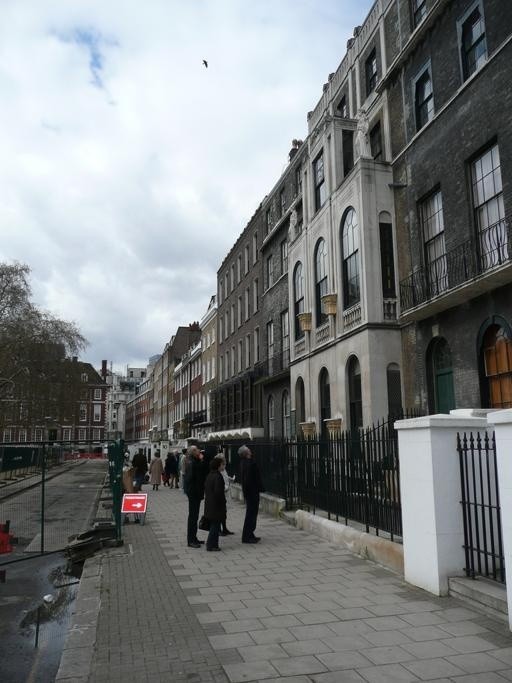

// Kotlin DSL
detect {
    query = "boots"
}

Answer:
[153,484,158,490]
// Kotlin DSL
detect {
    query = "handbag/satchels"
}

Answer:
[144,475,149,484]
[199,515,209,531]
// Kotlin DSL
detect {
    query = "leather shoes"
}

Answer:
[188,529,234,551]
[242,537,261,543]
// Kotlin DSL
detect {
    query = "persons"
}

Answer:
[238,445,262,543]
[182,444,206,548]
[214,451,235,537]
[123,446,207,499]
[200,458,226,551]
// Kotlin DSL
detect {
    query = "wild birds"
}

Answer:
[202,59,208,69]
